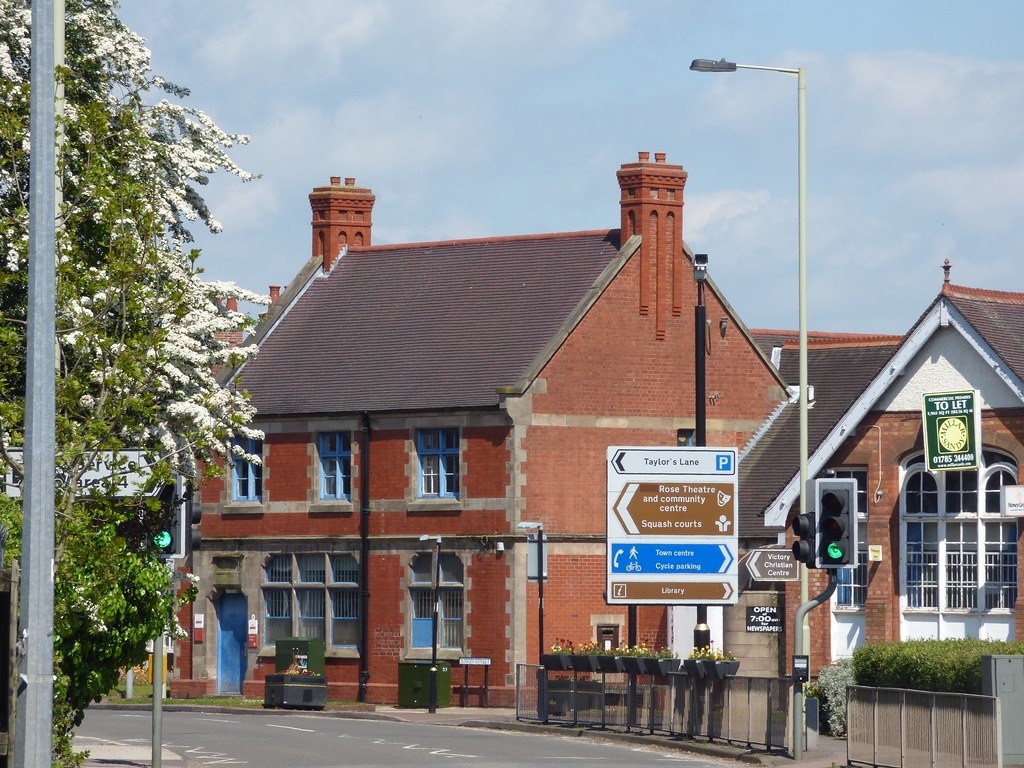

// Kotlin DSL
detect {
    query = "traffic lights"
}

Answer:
[793,511,815,570]
[814,477,859,569]
[181,492,202,557]
[143,469,185,558]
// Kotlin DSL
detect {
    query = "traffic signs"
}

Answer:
[745,548,799,581]
[605,479,737,536]
[611,580,733,601]
[606,540,734,575]
[3,446,172,504]
[610,446,736,476]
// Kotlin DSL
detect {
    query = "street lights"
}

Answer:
[688,252,711,653]
[691,57,809,690]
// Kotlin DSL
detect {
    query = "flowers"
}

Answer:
[545,637,737,662]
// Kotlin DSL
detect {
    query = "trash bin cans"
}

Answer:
[275,636,326,673]
[396,660,451,706]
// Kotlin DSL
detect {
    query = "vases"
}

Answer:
[539,652,742,681]
